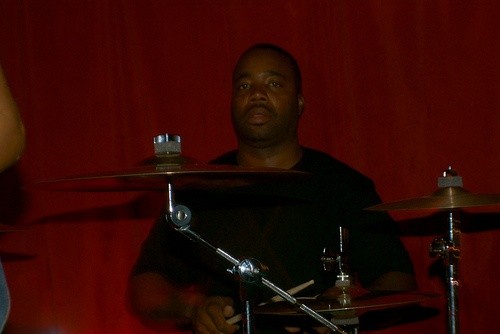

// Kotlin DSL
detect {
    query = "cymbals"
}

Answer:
[244,294,418,314]
[366,187,498,211]
[18,153,302,187]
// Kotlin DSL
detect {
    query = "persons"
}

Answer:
[127,42,417,333]
